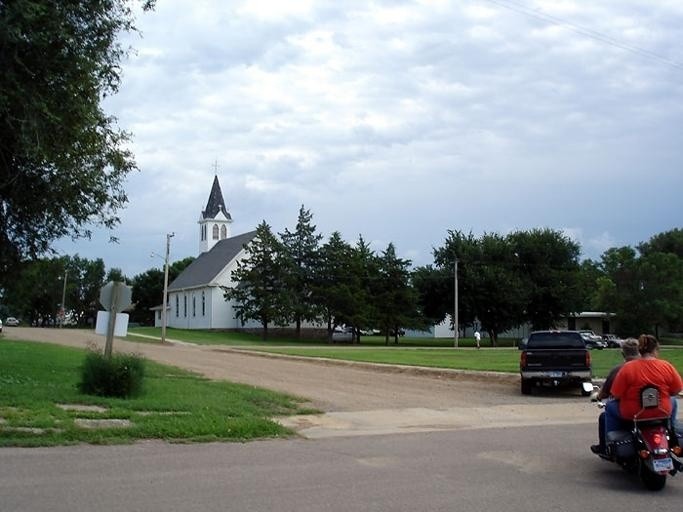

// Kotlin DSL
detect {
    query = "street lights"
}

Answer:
[58,276,67,327]
[150,252,169,344]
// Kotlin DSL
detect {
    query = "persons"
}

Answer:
[589,337,683,477]
[597,334,678,465]
[473,330,480,349]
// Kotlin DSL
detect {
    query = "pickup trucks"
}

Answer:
[519,330,592,397]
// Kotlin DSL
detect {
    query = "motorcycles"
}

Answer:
[582,381,682,492]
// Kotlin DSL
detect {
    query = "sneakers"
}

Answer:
[591,444,615,461]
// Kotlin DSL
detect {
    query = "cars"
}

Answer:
[334,325,405,338]
[5,317,18,327]
[0,319,2,332]
[579,330,626,349]
